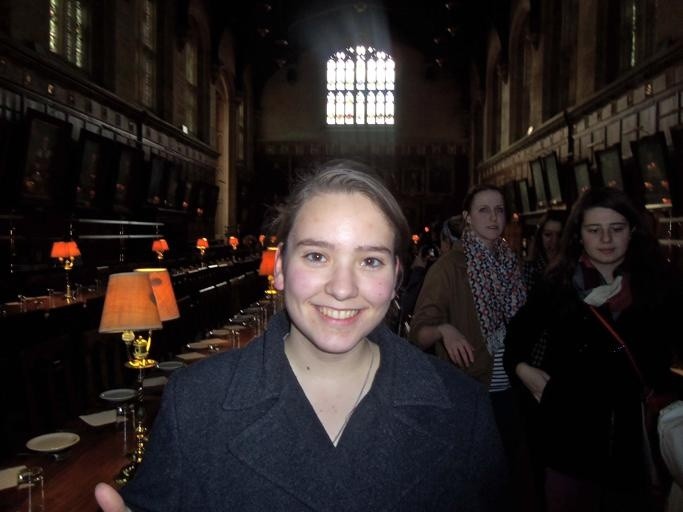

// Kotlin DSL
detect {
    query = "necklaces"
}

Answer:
[282,332,373,444]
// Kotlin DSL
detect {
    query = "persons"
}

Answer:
[96,157,506,510]
[409,184,530,396]
[392,207,572,338]
[502,185,676,510]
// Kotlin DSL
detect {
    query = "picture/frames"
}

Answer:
[12,107,182,215]
[506,123,683,213]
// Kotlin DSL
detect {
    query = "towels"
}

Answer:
[0,464,28,491]
[201,338,229,345]
[177,351,207,360]
[143,375,168,388]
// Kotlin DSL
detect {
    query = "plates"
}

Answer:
[100,387,135,402]
[141,378,171,388]
[27,430,81,451]
[0,466,31,490]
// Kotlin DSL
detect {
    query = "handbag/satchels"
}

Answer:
[644,394,679,435]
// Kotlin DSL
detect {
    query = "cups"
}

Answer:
[184,295,285,355]
[176,354,206,361]
[18,466,43,499]
[158,359,185,374]
[119,406,136,431]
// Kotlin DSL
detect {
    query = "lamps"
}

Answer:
[229,237,239,256]
[196,240,209,268]
[259,235,266,248]
[98,269,180,484]
[50,240,84,304]
[152,239,170,268]
